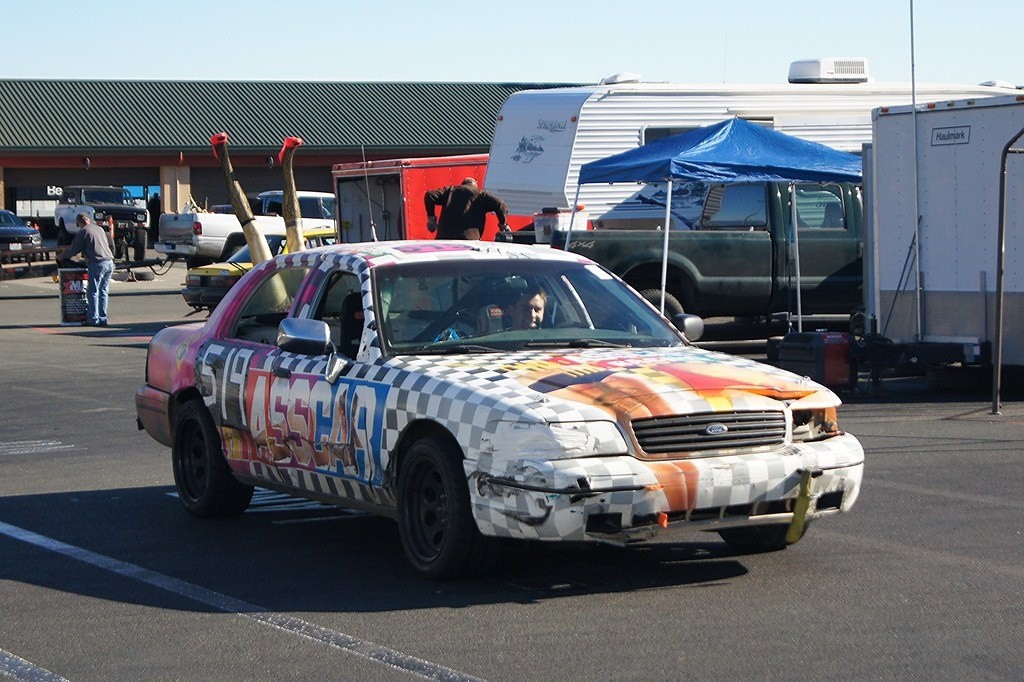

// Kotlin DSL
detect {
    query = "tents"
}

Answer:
[565,116,862,332]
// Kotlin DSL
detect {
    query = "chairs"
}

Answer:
[820,202,844,228]
[341,291,394,358]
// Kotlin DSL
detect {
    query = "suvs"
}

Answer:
[54,185,151,262]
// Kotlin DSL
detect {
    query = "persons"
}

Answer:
[210,133,311,313]
[505,283,547,331]
[55,212,114,325]
[147,193,160,231]
[424,178,512,240]
[340,292,393,361]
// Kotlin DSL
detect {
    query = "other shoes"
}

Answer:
[83,321,97,327]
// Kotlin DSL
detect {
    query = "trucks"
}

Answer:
[483,56,1024,229]
[861,94,1024,372]
[332,152,537,245]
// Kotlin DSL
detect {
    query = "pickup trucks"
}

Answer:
[550,178,866,337]
[153,189,336,272]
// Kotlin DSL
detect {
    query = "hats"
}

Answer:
[75,213,89,227]
[461,178,477,185]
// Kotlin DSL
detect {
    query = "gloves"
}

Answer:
[498,223,511,232]
[427,216,437,233]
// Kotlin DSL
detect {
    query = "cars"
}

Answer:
[180,227,339,320]
[0,209,43,261]
[136,240,866,583]
